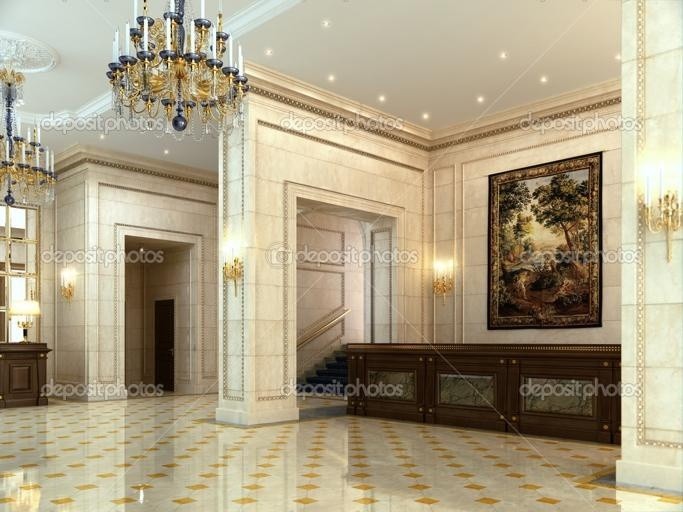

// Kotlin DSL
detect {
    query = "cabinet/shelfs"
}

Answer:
[345,343,622,445]
[0,342,52,409]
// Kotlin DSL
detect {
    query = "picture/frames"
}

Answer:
[487,152,602,330]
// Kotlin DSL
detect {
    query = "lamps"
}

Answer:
[0,30,57,204]
[643,166,683,261]
[60,268,73,306]
[7,300,41,343]
[433,262,453,305]
[106,0,249,130]
[222,243,242,297]
